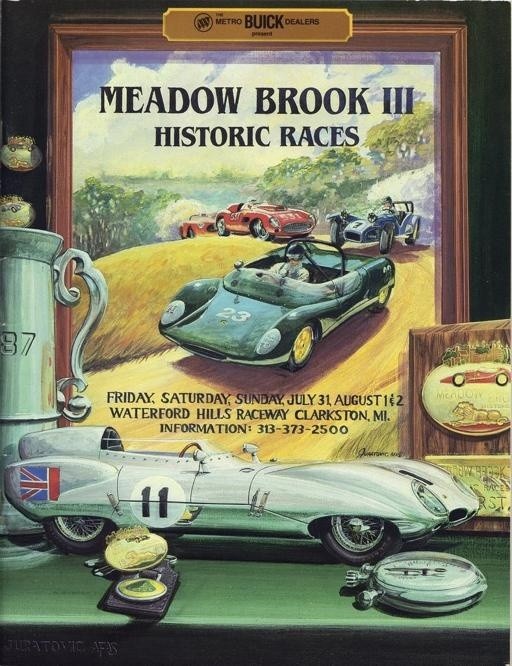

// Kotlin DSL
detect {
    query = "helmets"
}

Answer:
[379,196,392,204]
[286,244,303,260]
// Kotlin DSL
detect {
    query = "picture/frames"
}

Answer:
[40,8,471,563]
